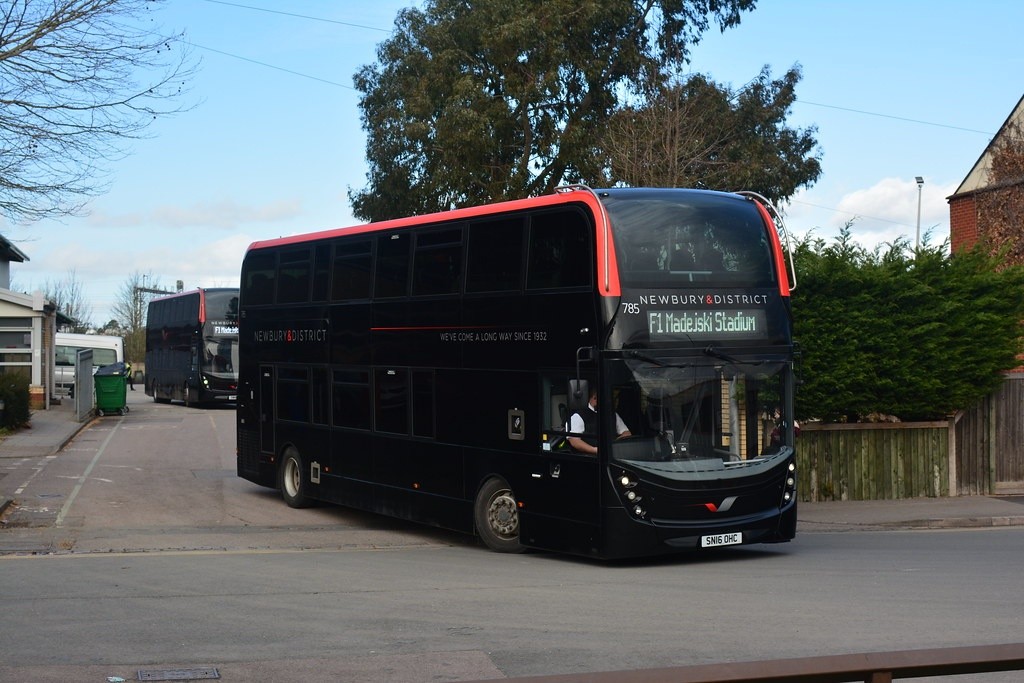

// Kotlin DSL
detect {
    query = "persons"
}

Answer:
[565,388,631,454]
[125,361,135,390]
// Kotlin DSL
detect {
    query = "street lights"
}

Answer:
[915,176,925,254]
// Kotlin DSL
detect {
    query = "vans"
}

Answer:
[56,332,124,385]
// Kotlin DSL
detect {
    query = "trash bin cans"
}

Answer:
[94,362,129,417]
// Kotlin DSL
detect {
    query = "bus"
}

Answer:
[145,287,240,406]
[237,185,796,560]
